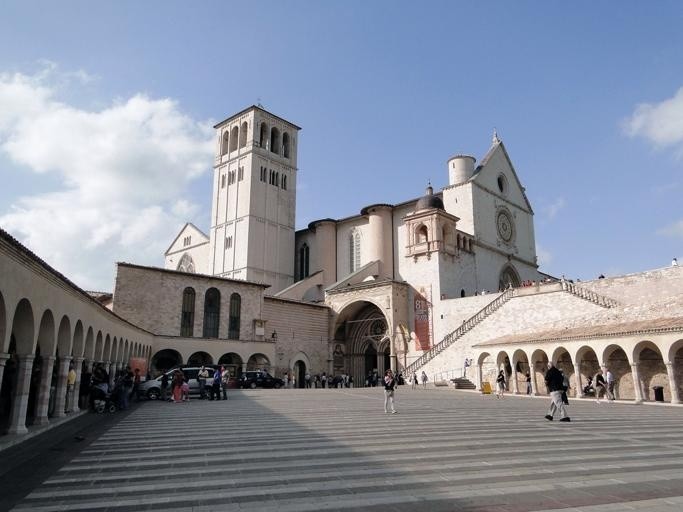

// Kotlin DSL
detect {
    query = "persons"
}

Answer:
[473,274,566,297]
[672,258,678,266]
[64,364,231,413]
[282,369,428,390]
[495,362,616,422]
[381,369,397,414]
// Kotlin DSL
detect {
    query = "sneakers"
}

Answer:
[545,415,553,421]
[560,418,570,421]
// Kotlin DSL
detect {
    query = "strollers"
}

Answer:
[94,377,121,414]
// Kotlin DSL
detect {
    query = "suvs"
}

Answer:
[234,370,284,389]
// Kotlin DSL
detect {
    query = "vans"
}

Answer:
[138,366,224,400]
